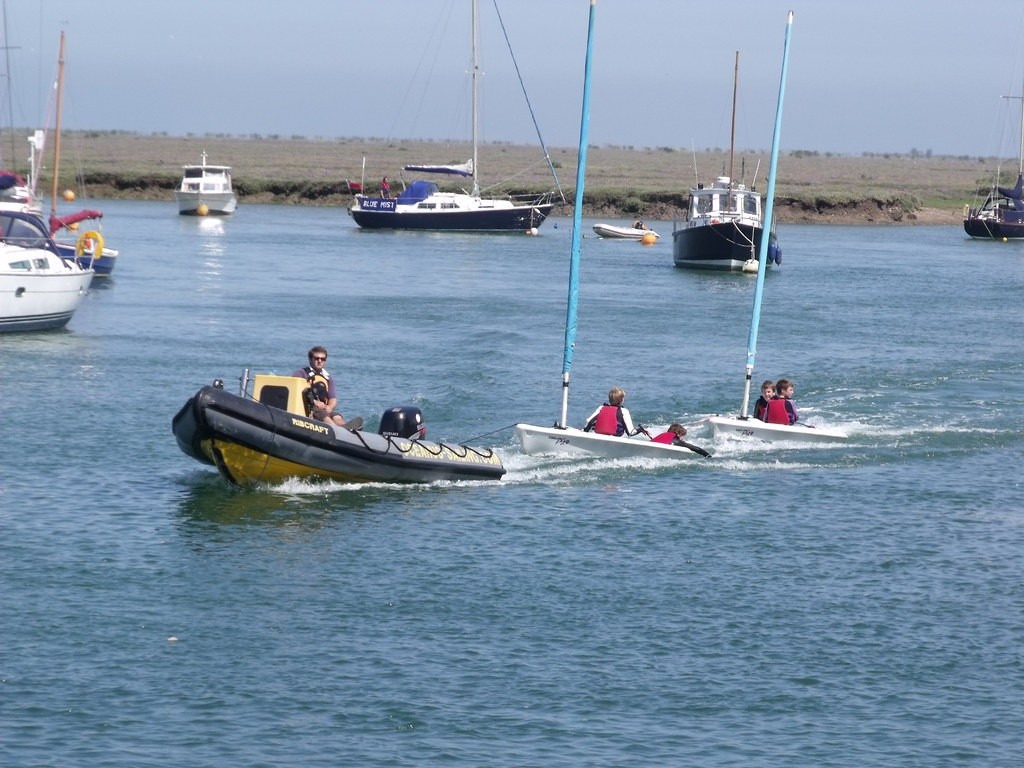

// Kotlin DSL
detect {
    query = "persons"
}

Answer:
[292,346,364,433]
[754,381,779,424]
[635,221,643,230]
[584,388,650,441]
[380,176,392,200]
[761,379,800,426]
[649,424,714,460]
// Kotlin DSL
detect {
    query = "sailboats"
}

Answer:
[705,7,851,449]
[514,0,715,463]
[963,59,1024,240]
[348,1,568,235]
[670,48,781,273]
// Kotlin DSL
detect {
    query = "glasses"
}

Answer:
[312,356,326,361]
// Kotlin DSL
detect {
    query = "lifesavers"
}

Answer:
[76,230,102,260]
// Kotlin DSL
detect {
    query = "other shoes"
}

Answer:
[341,417,363,430]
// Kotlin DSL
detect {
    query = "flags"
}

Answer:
[347,179,364,197]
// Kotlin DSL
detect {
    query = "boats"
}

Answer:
[0,0,117,332]
[592,223,661,242]
[171,368,505,491]
[173,150,237,216]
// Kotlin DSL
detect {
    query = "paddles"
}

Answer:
[795,421,815,429]
[639,424,653,441]
